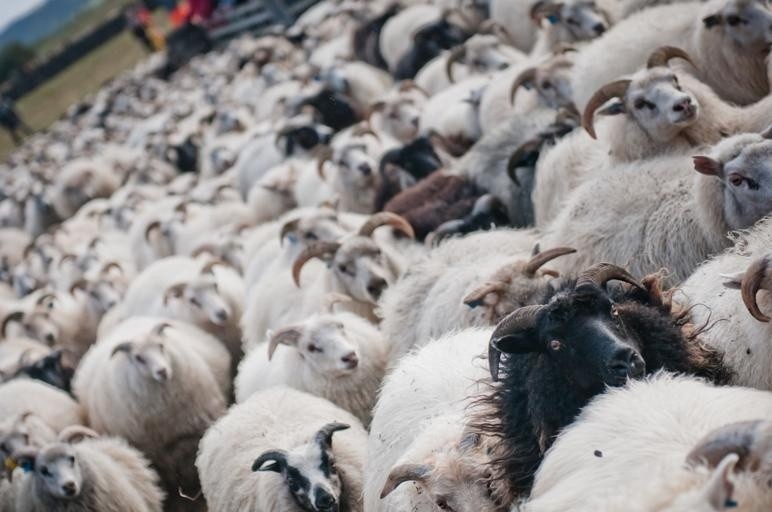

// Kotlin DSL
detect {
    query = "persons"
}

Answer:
[120,1,218,58]
[1,98,34,147]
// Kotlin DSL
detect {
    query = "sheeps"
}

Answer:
[0,0,772,512]
